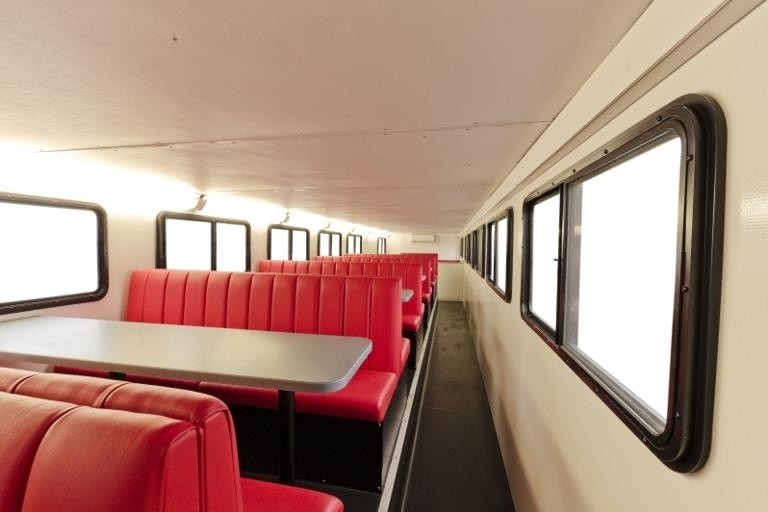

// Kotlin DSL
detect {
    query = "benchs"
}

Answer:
[55,267,400,492]
[2,391,201,511]
[2,366,345,512]
[259,253,437,370]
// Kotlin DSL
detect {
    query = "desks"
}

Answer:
[2,314,374,487]
